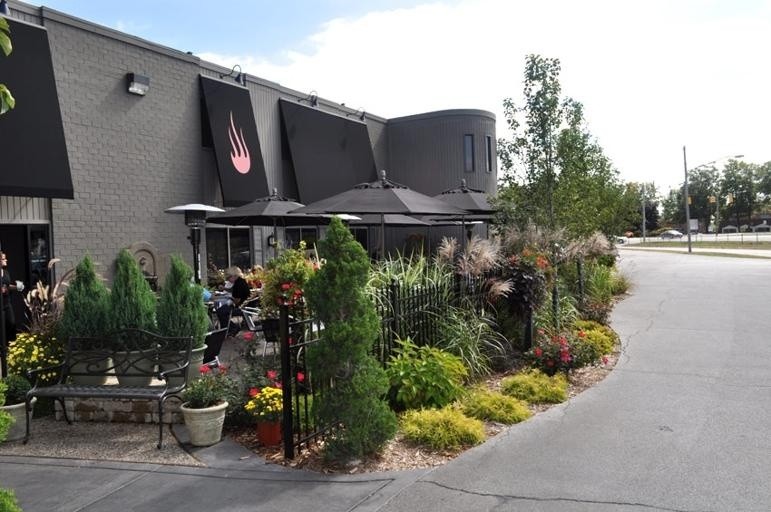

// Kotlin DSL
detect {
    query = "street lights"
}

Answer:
[641,180,685,246]
[683,146,746,253]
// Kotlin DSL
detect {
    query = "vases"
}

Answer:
[180,401,229,445]
[256,421,281,448]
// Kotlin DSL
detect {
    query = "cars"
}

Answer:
[623,231,635,238]
[607,235,628,244]
[660,229,683,240]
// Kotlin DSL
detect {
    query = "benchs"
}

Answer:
[23,329,193,449]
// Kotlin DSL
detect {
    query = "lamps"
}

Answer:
[125,71,151,96]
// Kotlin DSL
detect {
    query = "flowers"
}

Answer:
[183,362,231,406]
[245,386,285,418]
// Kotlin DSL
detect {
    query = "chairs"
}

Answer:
[198,291,314,364]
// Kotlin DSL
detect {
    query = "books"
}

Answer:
[286,169,516,263]
[205,186,363,264]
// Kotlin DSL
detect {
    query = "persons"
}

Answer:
[245,264,263,289]
[190,276,217,302]
[214,265,250,336]
[0,251,19,346]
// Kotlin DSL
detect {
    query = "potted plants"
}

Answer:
[155,254,207,386]
[0,377,37,441]
[58,256,109,385]
[103,249,158,388]
